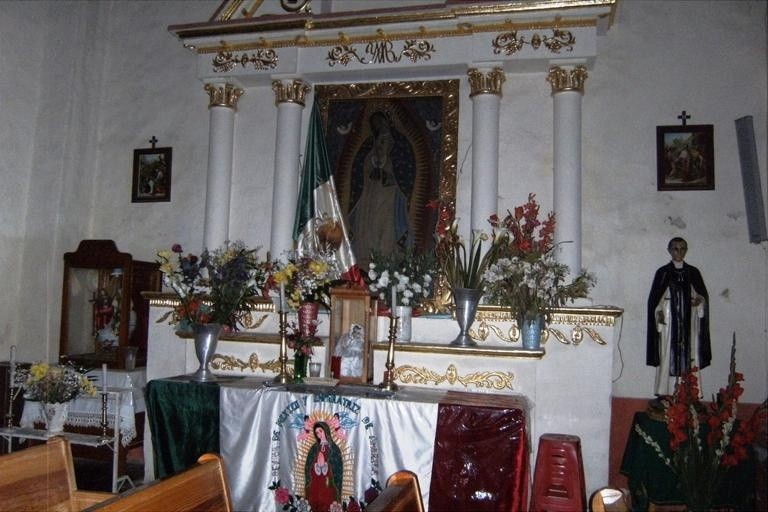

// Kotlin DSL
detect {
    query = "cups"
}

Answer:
[124,345,139,371]
[309,362,322,377]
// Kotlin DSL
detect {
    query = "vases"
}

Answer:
[520,312,541,349]
[393,306,412,341]
[451,290,478,346]
[296,300,319,336]
[294,350,303,384]
[41,402,69,437]
[191,324,219,383]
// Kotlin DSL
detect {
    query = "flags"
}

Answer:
[291,94,368,292]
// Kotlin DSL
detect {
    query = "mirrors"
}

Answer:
[58,239,134,369]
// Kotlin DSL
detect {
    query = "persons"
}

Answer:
[336,324,363,377]
[304,420,343,510]
[141,168,165,195]
[645,236,713,401]
[297,414,310,440]
[668,142,694,183]
[327,413,346,440]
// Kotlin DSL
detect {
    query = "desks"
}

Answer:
[20,366,146,443]
[146,374,532,512]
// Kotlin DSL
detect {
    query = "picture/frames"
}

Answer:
[657,123,715,191]
[132,146,172,203]
[314,78,462,289]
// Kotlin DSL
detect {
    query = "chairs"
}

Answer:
[79,453,235,511]
[362,470,426,511]
[0,435,82,512]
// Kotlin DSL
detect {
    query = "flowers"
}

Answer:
[274,249,345,315]
[490,193,597,329]
[368,249,435,306]
[283,319,323,356]
[426,198,493,290]
[25,362,97,402]
[157,240,278,333]
[636,333,768,512]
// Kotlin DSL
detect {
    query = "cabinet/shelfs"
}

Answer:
[0,383,136,494]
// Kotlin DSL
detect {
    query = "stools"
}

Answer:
[530,432,587,511]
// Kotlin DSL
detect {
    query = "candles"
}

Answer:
[10,345,16,385]
[281,279,286,314]
[391,285,396,316]
[102,364,107,393]
